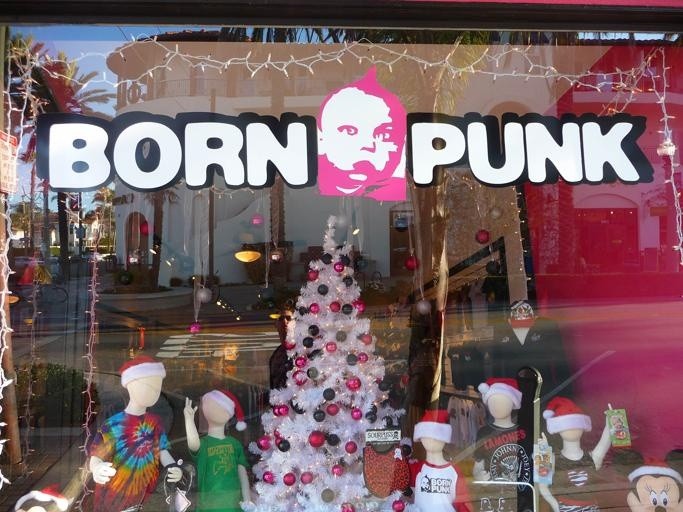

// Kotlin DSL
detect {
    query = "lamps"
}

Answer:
[234,243,262,263]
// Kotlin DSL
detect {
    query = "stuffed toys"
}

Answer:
[614,446,682,511]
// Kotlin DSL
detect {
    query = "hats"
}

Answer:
[543,396,592,435]
[120,355,167,389]
[478,377,523,410]
[202,388,247,432]
[413,410,453,444]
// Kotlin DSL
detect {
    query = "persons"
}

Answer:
[489,300,574,511]
[404,410,473,512]
[317,64,407,201]
[87,353,183,511]
[538,395,614,511]
[472,377,531,511]
[183,387,251,512]
